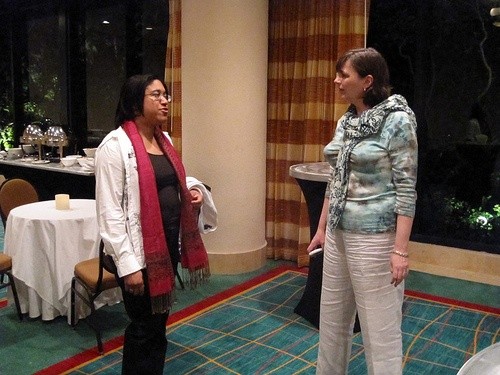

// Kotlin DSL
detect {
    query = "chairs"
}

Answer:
[0,178,40,234]
[70,239,128,357]
[0,250,24,325]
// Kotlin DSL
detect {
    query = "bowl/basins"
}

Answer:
[83,148,97,158]
[8,148,22,158]
[22,145,36,154]
[66,155,82,163]
[60,158,76,167]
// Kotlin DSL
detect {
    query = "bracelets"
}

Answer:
[393,249,409,258]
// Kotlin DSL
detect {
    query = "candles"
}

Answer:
[55,194,69,210]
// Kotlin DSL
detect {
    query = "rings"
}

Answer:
[129,289,134,293]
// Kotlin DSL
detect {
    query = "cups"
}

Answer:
[308,248,323,270]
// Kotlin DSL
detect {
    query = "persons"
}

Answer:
[92,75,203,375]
[306,49,418,375]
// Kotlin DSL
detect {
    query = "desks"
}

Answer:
[289,161,362,335]
[3,199,126,327]
[0,151,97,202]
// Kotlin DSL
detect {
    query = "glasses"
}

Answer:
[144,92,172,103]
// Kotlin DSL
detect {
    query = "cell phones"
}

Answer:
[309,248,322,258]
